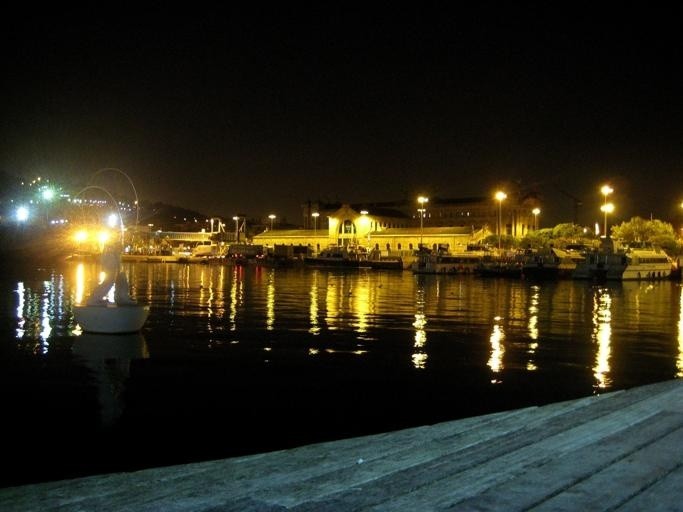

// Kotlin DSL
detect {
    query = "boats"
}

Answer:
[67,237,682,281]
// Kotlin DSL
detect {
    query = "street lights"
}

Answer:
[269,215,276,230]
[418,196,429,250]
[311,212,319,237]
[231,216,240,245]
[496,191,506,249]
[533,209,540,229]
[599,184,615,236]
